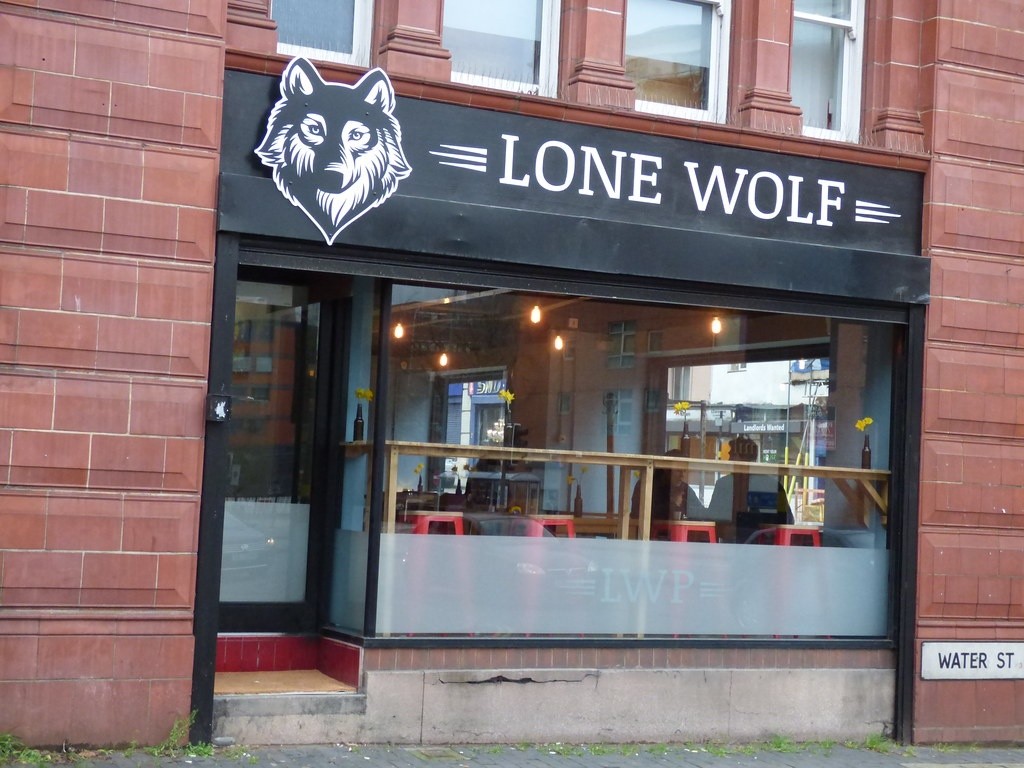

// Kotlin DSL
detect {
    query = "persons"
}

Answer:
[706,436,794,546]
[629,449,708,520]
[466,458,502,512]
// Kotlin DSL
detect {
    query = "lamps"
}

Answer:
[555,328,563,350]
[530,305,542,324]
[394,318,404,339]
[711,313,722,335]
[439,349,448,367]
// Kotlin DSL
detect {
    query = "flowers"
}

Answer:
[567,466,587,486]
[854,415,873,433]
[355,386,372,403]
[499,390,514,410]
[415,463,424,475]
[464,465,470,471]
[674,401,691,420]
[452,467,458,480]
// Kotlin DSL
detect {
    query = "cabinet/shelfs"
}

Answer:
[226,316,303,505]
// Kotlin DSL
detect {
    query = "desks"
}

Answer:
[395,490,441,522]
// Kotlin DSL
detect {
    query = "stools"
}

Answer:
[399,511,821,547]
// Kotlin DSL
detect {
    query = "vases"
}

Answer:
[418,475,424,492]
[503,411,514,448]
[861,434,872,469]
[353,403,364,442]
[455,479,462,494]
[681,421,691,458]
[573,484,583,518]
